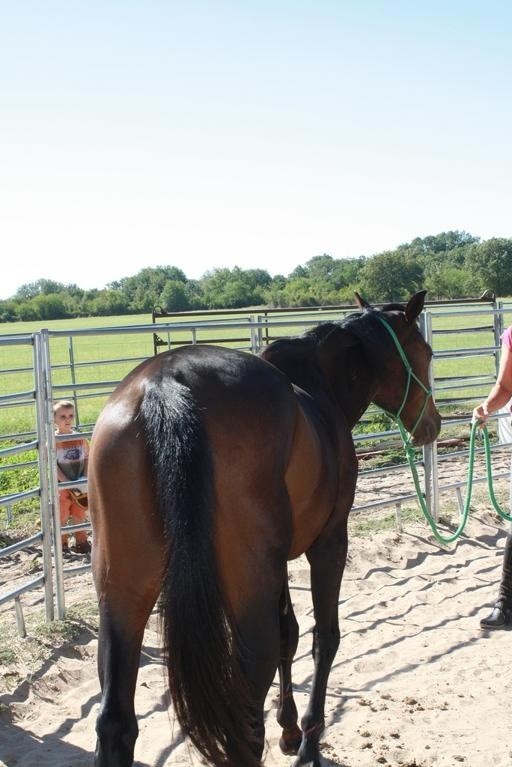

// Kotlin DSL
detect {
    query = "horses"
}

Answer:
[87,291,441,767]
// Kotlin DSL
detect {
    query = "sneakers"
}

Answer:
[75,541,95,555]
[59,543,72,560]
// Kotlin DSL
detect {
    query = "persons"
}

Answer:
[44,400,93,554]
[471,325,512,629]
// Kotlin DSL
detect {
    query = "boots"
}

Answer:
[479,533,511,631]
